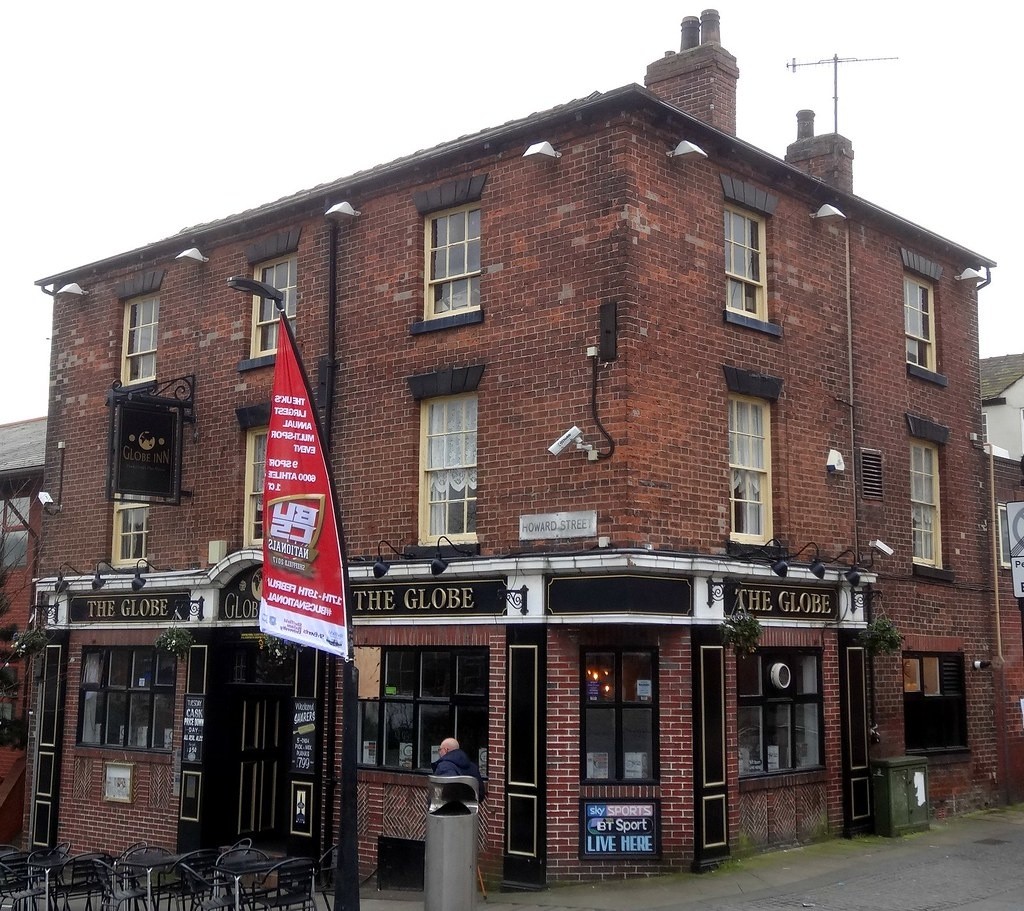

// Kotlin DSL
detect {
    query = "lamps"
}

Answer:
[55,282,90,296]
[521,140,562,162]
[54,563,86,594]
[954,267,987,284]
[666,140,708,162]
[431,536,474,575]
[785,542,826,580]
[373,540,415,579]
[174,248,210,265]
[809,204,846,224]
[131,559,173,592]
[830,549,861,588]
[758,538,788,579]
[91,560,124,592]
[322,201,362,221]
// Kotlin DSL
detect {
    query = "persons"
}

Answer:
[431,738,485,804]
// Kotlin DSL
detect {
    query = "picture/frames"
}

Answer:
[290,781,313,837]
[102,761,135,804]
[179,770,202,822]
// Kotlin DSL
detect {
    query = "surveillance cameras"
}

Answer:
[38,492,54,508]
[869,540,894,557]
[549,427,582,456]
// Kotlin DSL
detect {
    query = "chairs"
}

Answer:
[0,837,338,911]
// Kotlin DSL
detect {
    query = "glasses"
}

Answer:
[438,748,445,751]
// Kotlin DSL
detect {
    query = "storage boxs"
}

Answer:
[164,728,173,749]
[738,748,751,775]
[398,743,413,770]
[625,752,649,779]
[94,724,100,743]
[118,726,124,744]
[768,745,779,770]
[432,746,442,763]
[478,748,488,777]
[587,752,610,779]
[363,740,376,765]
[586,681,600,700]
[137,727,148,747]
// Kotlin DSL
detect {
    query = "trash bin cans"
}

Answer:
[423,776,479,909]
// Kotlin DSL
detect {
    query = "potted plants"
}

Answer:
[717,612,764,665]
[857,617,903,661]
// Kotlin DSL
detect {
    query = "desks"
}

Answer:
[210,857,316,911]
[23,852,108,911]
[118,852,216,911]
